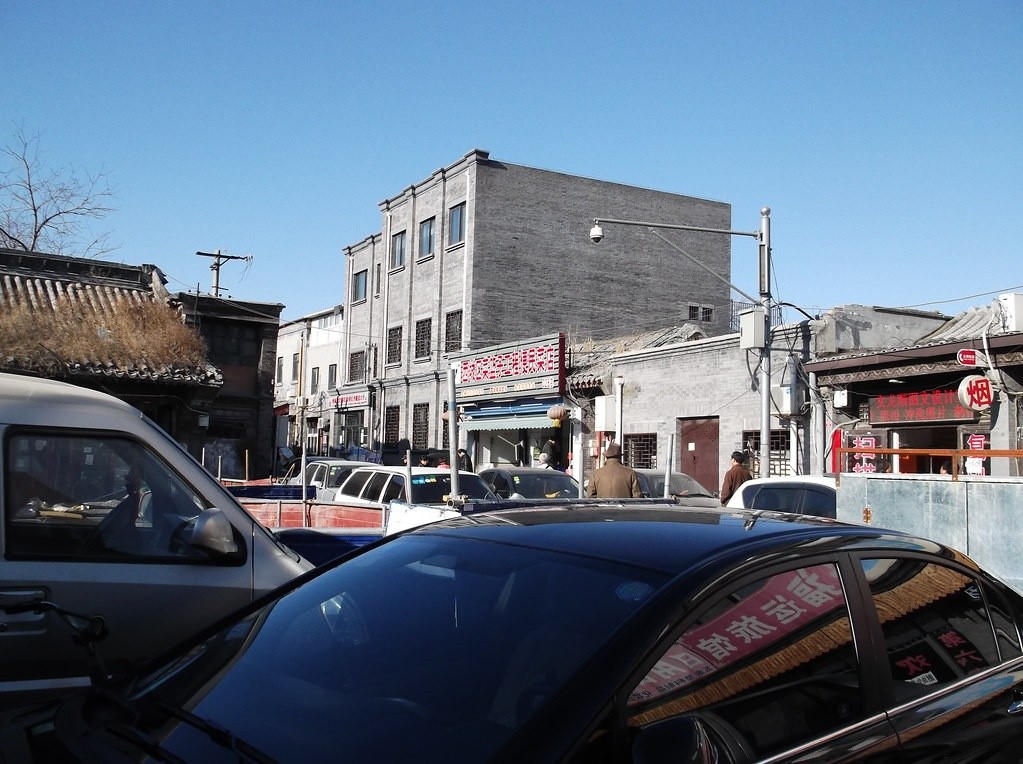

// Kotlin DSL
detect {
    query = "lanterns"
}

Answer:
[441,409,458,424]
[547,404,566,427]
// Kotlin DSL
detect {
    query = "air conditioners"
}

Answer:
[317,417,330,428]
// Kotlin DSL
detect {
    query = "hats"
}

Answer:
[602,443,624,458]
[402,455,407,459]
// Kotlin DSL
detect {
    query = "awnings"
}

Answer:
[459,415,555,431]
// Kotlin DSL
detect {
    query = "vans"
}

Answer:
[0,372,370,743]
[335,464,507,520]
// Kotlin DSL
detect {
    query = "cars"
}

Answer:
[581,469,723,508]
[287,459,378,503]
[284,454,349,478]
[720,472,839,521]
[478,466,590,504]
[0,483,1022,763]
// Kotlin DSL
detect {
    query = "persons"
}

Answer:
[402,455,412,467]
[418,456,431,467]
[587,443,644,498]
[940,461,960,475]
[437,457,450,469]
[874,459,891,473]
[721,451,752,507]
[537,437,559,471]
[457,448,473,473]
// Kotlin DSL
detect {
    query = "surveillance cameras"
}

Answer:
[590,226,604,243]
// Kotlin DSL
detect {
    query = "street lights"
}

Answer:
[587,207,776,481]
